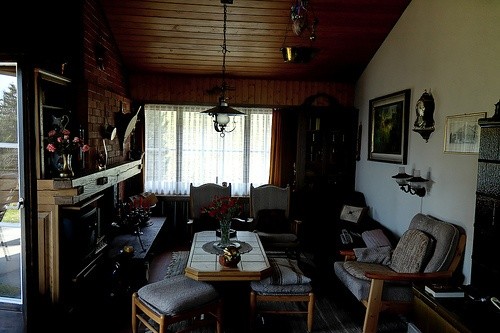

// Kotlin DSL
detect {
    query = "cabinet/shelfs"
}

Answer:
[60,204,108,282]
[290,94,358,252]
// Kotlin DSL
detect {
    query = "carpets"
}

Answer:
[146,250,364,333]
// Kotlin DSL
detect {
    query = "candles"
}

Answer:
[129,197,149,211]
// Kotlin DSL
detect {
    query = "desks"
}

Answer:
[111,216,167,284]
[184,230,271,328]
[409,283,500,333]
[36,160,143,309]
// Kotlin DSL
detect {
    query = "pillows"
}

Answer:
[388,229,430,273]
[259,260,312,285]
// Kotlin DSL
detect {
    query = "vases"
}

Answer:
[59,153,74,180]
[217,220,236,248]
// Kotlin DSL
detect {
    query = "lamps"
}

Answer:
[280,0,319,64]
[391,167,430,213]
[101,117,117,141]
[200,0,249,138]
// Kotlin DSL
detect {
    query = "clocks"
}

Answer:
[412,88,435,143]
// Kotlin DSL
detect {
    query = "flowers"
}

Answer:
[200,193,243,246]
[45,129,90,155]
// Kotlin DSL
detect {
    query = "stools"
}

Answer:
[131,275,313,333]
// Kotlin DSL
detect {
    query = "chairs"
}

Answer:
[333,210,466,333]
[190,183,303,259]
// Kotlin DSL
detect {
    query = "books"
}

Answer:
[424,284,465,297]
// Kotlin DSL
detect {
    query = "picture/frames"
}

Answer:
[442,112,487,154]
[367,89,411,166]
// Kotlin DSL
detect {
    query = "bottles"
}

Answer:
[99,139,107,170]
